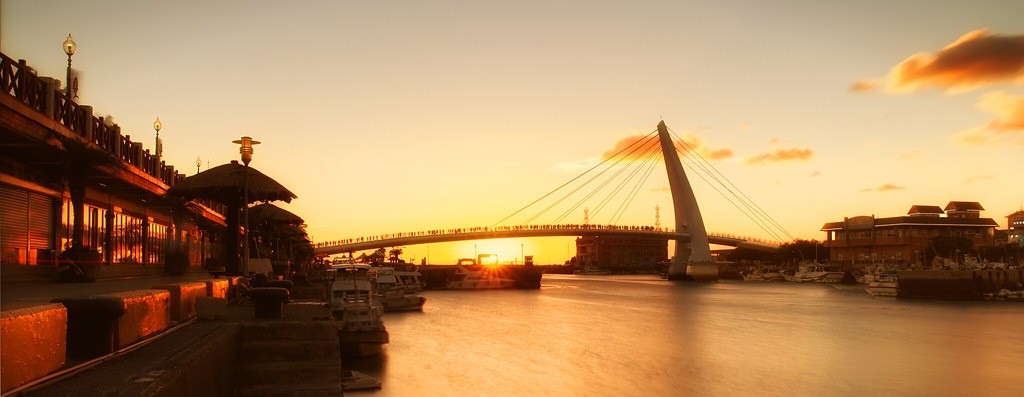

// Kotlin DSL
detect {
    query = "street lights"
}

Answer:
[64,32,73,128]
[154,117,162,154]
[232,136,263,279]
[196,157,201,174]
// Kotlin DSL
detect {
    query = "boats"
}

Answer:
[445,263,516,290]
[318,256,427,359]
[736,241,1024,303]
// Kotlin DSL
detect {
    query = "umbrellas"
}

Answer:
[167,161,296,272]
[238,202,315,264]
